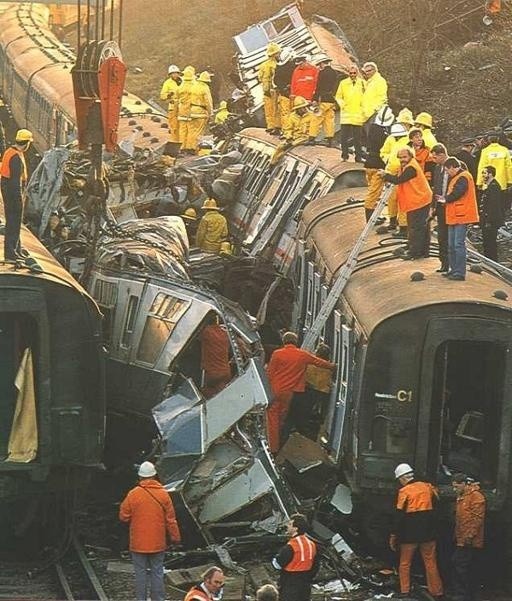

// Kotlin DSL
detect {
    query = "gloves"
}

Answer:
[464,535,475,550]
[389,532,398,554]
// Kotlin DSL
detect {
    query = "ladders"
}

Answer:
[299,183,395,352]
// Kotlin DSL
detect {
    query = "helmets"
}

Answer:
[414,113,432,128]
[198,70,212,83]
[201,197,220,210]
[184,69,194,80]
[292,95,309,110]
[267,42,280,56]
[181,208,197,221]
[167,64,180,74]
[393,463,413,479]
[137,460,157,478]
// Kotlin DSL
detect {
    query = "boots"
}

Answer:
[387,217,407,237]
[365,208,386,225]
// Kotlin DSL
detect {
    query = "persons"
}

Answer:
[196,194,229,260]
[0,129,35,263]
[483,0,511,30]
[269,514,320,601]
[254,584,280,601]
[388,463,446,601]
[195,310,245,401]
[258,330,339,459]
[182,566,226,601]
[293,342,331,443]
[160,64,230,153]
[117,461,180,601]
[180,207,198,246]
[256,41,512,283]
[450,469,488,597]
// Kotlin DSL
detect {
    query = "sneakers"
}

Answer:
[4,253,27,262]
[265,128,284,139]
[436,269,449,272]
[355,157,365,164]
[401,253,422,260]
[341,155,350,163]
[442,273,452,277]
[306,139,332,147]
[449,275,465,280]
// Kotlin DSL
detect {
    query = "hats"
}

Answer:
[315,54,332,65]
[294,56,307,64]
[375,106,394,127]
[15,129,34,141]
[396,107,414,126]
[277,50,293,65]
[390,123,407,137]
